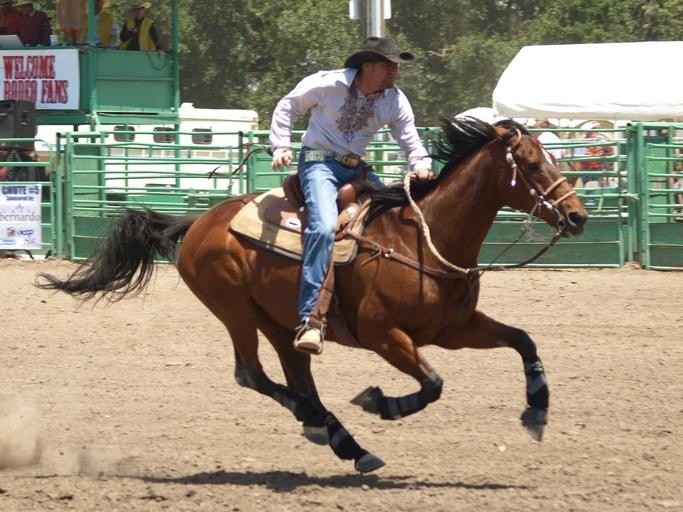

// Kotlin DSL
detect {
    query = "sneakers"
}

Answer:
[296,326,322,353]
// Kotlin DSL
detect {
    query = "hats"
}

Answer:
[344,35,416,68]
[126,0,152,9]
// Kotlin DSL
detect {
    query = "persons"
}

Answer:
[118,0,166,59]
[535,119,614,188]
[269,37,433,354]
[5,0,50,47]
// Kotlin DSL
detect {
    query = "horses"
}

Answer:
[30,110,591,475]
